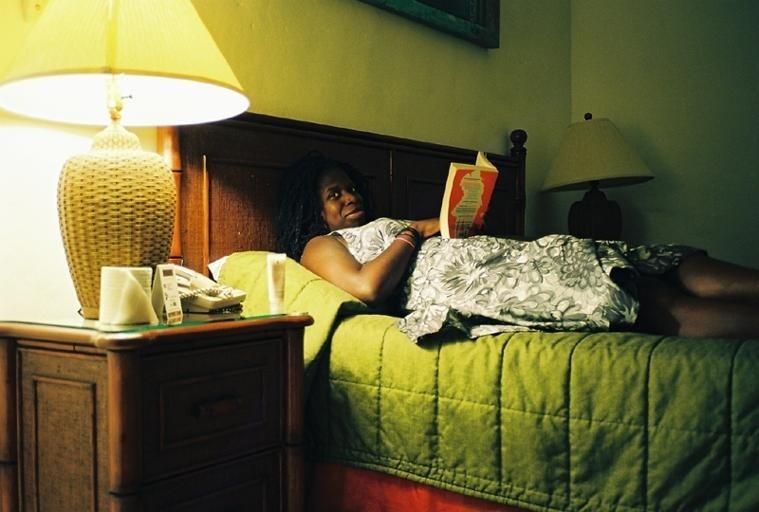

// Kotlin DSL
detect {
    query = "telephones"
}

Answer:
[166,264,247,313]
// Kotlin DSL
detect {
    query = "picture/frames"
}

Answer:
[368,0,499,49]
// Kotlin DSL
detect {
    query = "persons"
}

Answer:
[299,159,759,345]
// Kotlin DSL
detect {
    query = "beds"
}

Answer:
[154,106,758,510]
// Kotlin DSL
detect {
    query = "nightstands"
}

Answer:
[1,311,318,511]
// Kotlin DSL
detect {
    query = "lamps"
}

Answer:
[537,110,659,239]
[0,1,251,319]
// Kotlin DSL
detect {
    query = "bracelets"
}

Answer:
[395,227,424,252]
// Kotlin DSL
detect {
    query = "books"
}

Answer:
[439,151,499,239]
